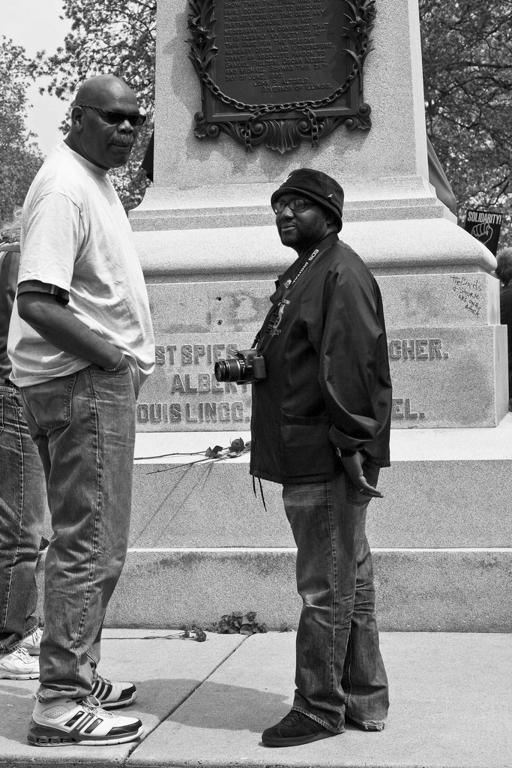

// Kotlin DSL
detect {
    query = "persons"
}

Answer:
[7,76,156,747]
[0,214,59,678]
[213,168,392,748]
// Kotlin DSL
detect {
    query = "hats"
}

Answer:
[271,168,343,231]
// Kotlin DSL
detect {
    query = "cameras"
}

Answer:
[215,349,267,385]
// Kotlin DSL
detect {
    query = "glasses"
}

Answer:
[272,199,313,213]
[82,104,146,126]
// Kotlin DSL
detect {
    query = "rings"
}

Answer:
[358,487,368,496]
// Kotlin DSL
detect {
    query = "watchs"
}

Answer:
[334,446,356,459]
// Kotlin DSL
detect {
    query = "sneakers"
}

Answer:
[90,675,137,708]
[28,694,143,747]
[261,709,333,746]
[0,629,44,680]
